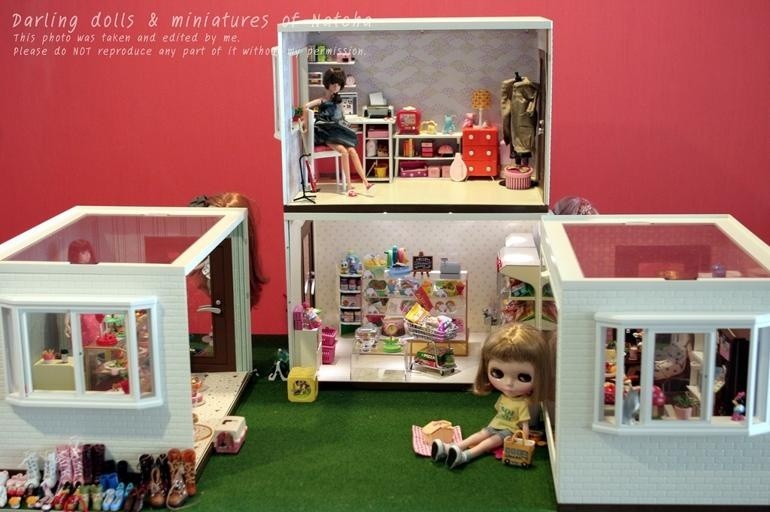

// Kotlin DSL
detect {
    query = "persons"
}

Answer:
[0,190,769,512]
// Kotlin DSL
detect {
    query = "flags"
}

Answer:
[0,190,770,512]
[290,42,539,205]
